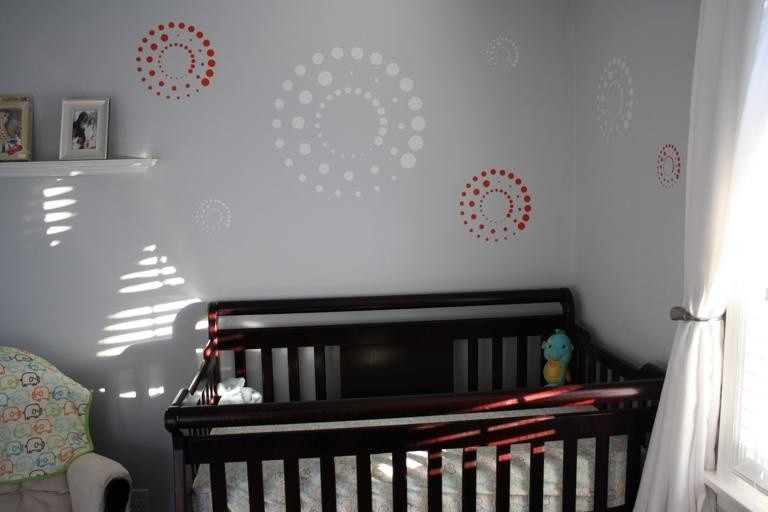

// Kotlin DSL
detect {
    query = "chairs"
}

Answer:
[0,344,132,512]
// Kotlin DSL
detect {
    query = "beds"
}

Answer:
[165,286,668,512]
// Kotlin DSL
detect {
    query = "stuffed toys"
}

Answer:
[537,329,574,389]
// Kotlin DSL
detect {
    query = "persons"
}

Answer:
[72,110,97,147]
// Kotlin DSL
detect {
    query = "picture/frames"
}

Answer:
[57,96,111,161]
[0,92,32,161]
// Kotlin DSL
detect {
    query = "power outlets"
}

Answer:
[131,489,150,512]
